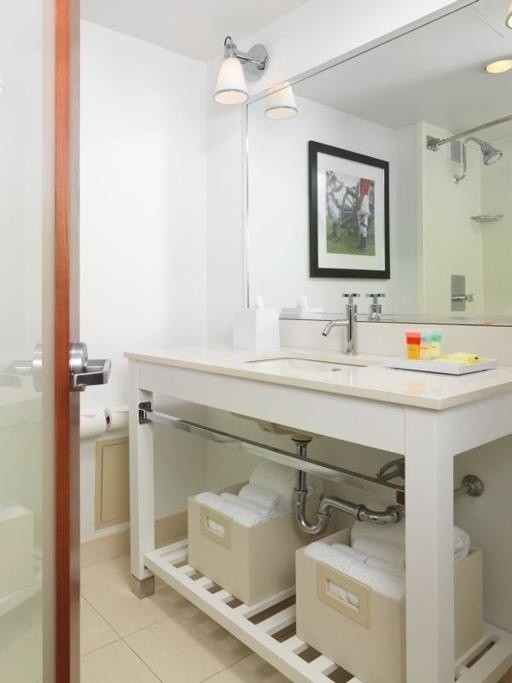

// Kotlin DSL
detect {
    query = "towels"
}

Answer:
[304,497,472,609]
[191,463,324,536]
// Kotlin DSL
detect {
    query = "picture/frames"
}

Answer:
[307,139,391,280]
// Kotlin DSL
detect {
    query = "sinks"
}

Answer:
[242,356,368,380]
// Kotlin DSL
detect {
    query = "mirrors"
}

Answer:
[239,0,512,328]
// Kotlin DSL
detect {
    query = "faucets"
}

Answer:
[321,292,360,355]
[364,292,386,321]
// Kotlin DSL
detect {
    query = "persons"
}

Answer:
[357,206,370,250]
[327,192,340,238]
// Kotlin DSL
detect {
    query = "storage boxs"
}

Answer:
[188,481,334,607]
[294,524,484,683]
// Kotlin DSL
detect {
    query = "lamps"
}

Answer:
[264,87,299,120]
[212,34,270,106]
